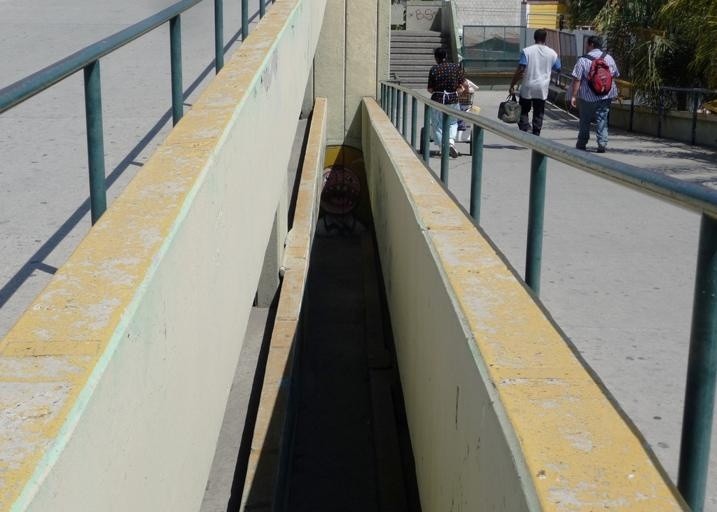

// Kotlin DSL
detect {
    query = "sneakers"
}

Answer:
[449,144,458,158]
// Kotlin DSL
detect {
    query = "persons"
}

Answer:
[508,28,563,136]
[564,35,621,153]
[457,66,479,142]
[427,46,470,158]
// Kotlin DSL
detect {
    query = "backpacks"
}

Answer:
[578,50,612,96]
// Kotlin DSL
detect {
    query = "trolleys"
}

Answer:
[421,78,478,152]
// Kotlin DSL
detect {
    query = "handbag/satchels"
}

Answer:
[497,93,521,123]
